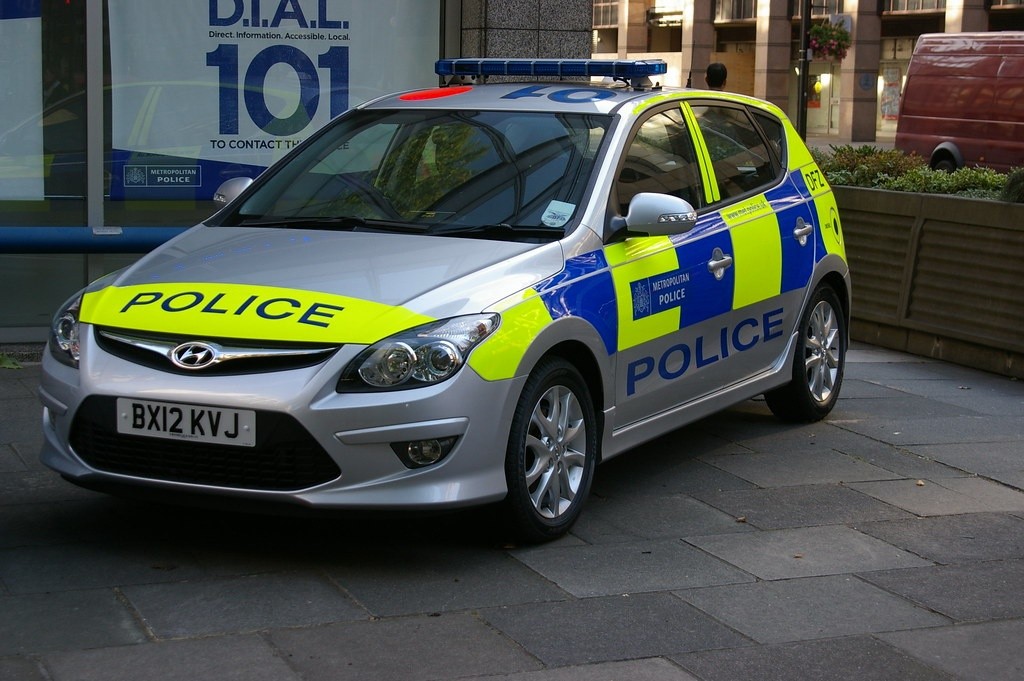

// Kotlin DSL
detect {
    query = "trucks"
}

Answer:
[895,32,1023,180]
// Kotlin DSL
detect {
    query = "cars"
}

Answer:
[38,54,856,545]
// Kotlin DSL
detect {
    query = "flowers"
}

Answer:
[807,25,852,60]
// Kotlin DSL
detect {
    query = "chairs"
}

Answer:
[442,135,745,233]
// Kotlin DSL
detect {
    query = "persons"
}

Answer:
[700,62,728,93]
